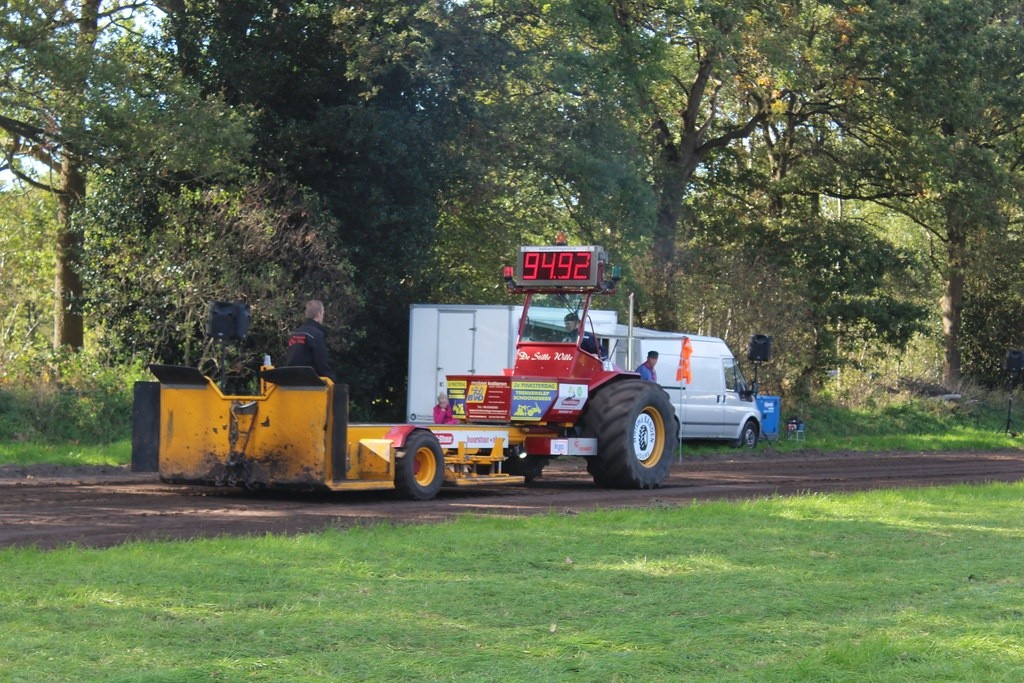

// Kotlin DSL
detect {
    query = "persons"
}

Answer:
[286,301,335,380]
[636,350,658,382]
[561,313,607,361]
[433,391,458,424]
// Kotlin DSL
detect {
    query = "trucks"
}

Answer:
[404,304,620,426]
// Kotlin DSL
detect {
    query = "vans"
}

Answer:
[620,327,771,454]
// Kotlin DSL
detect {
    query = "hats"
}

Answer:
[564,313,579,321]
[648,351,658,359]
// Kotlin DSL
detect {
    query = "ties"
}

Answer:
[642,361,655,381]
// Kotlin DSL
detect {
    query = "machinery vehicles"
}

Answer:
[123,235,681,503]
